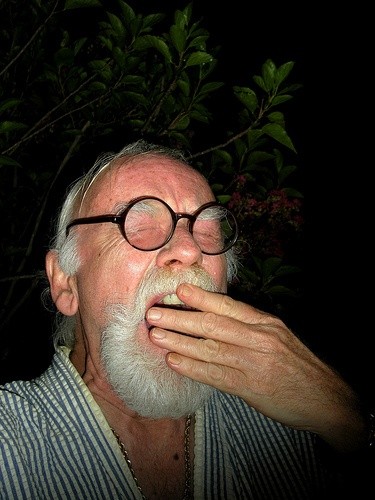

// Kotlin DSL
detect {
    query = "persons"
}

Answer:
[0,138,375,500]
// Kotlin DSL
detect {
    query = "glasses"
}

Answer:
[65,196,241,256]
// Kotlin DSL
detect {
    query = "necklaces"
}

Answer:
[110,414,193,500]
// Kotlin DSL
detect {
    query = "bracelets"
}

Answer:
[367,413,375,448]
